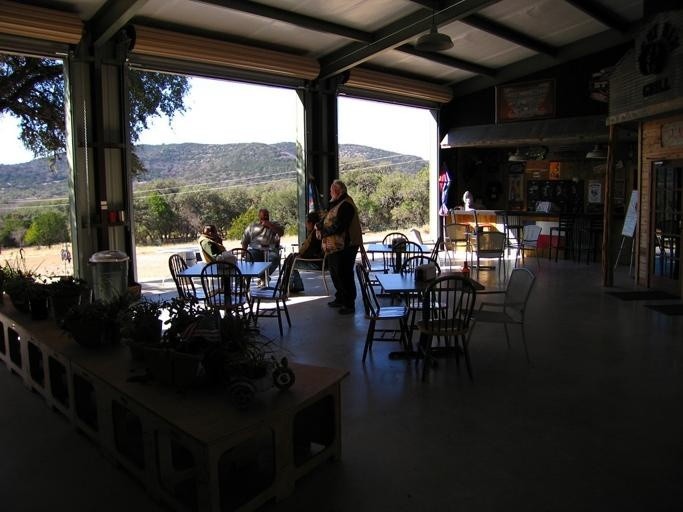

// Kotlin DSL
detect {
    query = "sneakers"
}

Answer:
[257,279,261,285]
[261,277,271,286]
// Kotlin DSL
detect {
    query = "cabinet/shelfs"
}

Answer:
[527,181,582,212]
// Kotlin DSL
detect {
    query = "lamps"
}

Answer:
[586,145,610,159]
[103,209,127,250]
[416,16,454,51]
[508,149,527,163]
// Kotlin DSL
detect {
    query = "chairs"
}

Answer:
[549,211,575,262]
[396,255,452,341]
[359,244,389,285]
[355,263,413,366]
[392,243,424,277]
[510,225,542,268]
[421,239,442,272]
[464,268,536,363]
[241,245,283,277]
[417,276,477,382]
[287,244,330,297]
[200,259,248,336]
[472,231,506,277]
[200,244,223,286]
[443,224,470,258]
[580,211,607,261]
[246,253,296,338]
[448,210,493,233]
[501,204,525,246]
[382,232,411,271]
[216,248,254,306]
[167,254,214,318]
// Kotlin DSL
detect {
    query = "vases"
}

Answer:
[215,349,247,371]
[124,322,162,348]
[10,292,30,315]
[165,346,201,372]
[53,290,82,318]
[28,291,49,315]
[191,330,220,347]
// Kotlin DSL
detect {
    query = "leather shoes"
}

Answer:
[328,300,346,307]
[338,307,355,314]
[292,288,304,292]
[289,289,293,291]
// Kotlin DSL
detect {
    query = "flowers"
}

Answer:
[128,292,264,339]
[0,258,89,290]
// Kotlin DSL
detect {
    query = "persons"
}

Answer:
[198,225,236,264]
[315,180,362,315]
[239,209,283,285]
[280,213,325,292]
